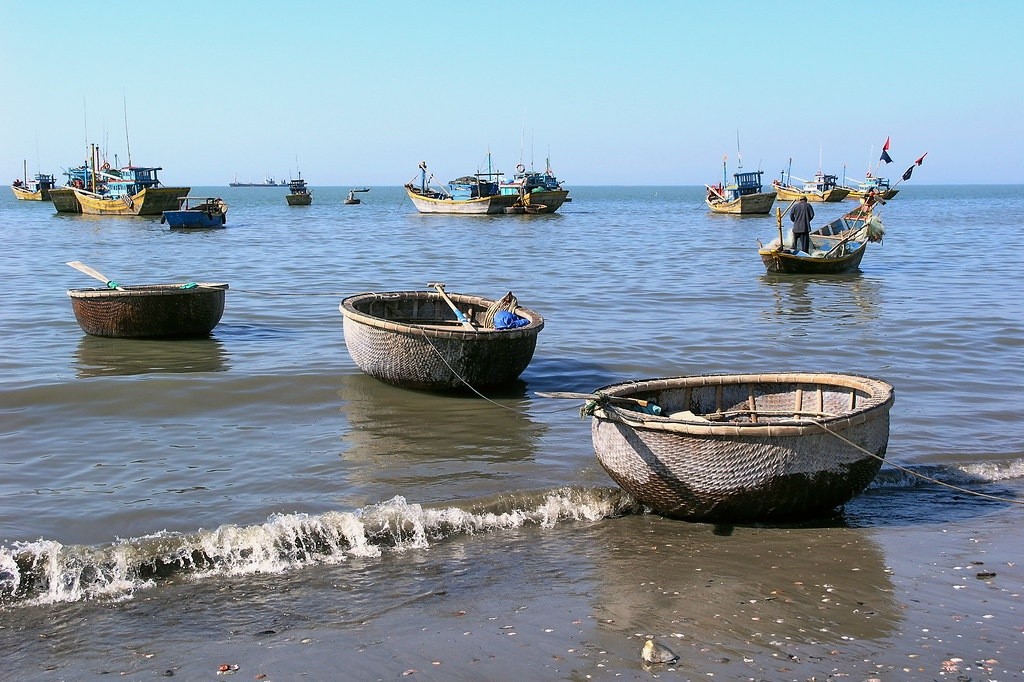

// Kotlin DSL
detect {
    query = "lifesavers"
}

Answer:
[103,164,110,170]
[516,164,526,174]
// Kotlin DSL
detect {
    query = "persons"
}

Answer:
[790,196,814,254]
[349,189,354,200]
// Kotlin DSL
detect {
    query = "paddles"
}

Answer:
[428,282,475,328]
[66,260,126,290]
[535,390,658,409]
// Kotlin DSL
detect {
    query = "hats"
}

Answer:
[799,196,808,200]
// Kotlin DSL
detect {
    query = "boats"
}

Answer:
[338,290,544,395]
[344,196,360,205]
[73,144,191,216]
[705,131,777,214]
[354,188,370,192]
[228,173,278,187]
[403,120,572,214]
[66,283,230,338]
[47,99,120,213]
[162,196,228,228]
[840,163,900,200]
[286,172,313,206]
[757,188,887,274]
[9,160,57,201]
[279,180,289,187]
[771,158,850,202]
[582,370,895,522]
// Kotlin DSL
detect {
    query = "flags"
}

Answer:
[883,137,889,150]
[880,150,893,164]
[915,154,926,166]
[902,165,913,181]
